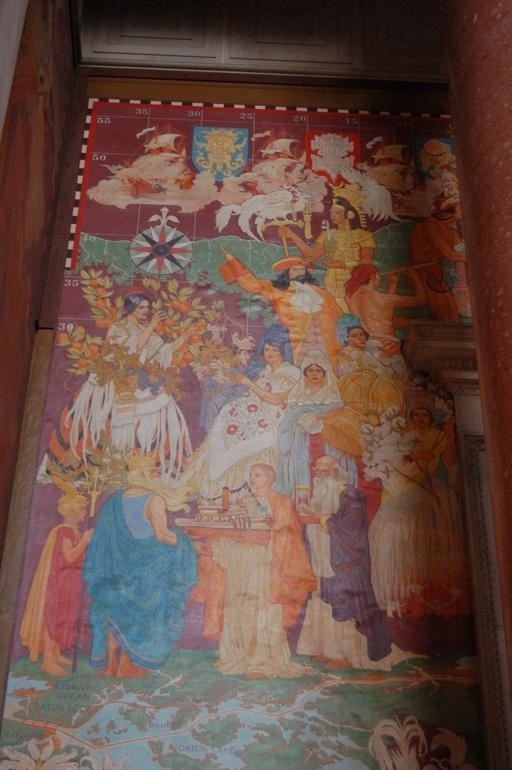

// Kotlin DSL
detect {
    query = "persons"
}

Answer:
[280,349,354,473]
[272,200,376,311]
[20,469,91,677]
[33,292,202,510]
[373,407,444,502]
[183,463,315,679]
[213,245,344,374]
[338,259,428,375]
[289,454,398,677]
[329,314,415,391]
[88,455,196,683]
[188,339,303,491]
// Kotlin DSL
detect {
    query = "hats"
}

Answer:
[334,311,361,346]
[271,255,315,275]
